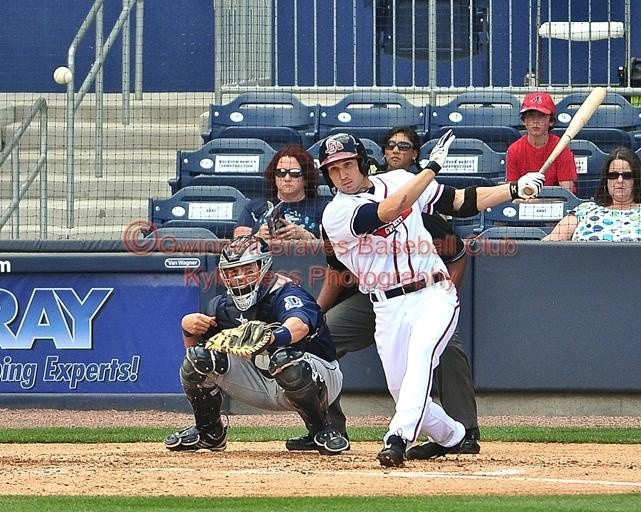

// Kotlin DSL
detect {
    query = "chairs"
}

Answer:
[371,0,490,87]
[136,86,640,248]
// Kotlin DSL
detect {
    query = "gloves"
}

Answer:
[427,129,455,171]
[516,173,545,199]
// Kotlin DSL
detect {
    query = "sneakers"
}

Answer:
[459,433,480,453]
[378,428,407,467]
[407,438,465,459]
[314,429,340,455]
[286,430,351,450]
[164,426,229,453]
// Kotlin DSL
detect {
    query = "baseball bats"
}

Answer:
[523,87,607,196]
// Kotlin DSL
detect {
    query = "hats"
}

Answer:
[519,93,554,115]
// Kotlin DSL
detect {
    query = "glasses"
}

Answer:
[274,168,303,177]
[606,172,633,179]
[385,141,411,151]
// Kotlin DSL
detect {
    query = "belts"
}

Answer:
[369,271,445,302]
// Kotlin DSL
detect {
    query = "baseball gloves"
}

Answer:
[203,319,273,357]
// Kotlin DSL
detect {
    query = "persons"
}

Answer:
[540,146,641,242]
[504,92,577,204]
[232,145,330,240]
[316,132,546,466]
[286,208,482,454]
[163,236,350,456]
[379,125,423,176]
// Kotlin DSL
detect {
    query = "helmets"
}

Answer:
[318,133,388,196]
[218,236,274,312]
